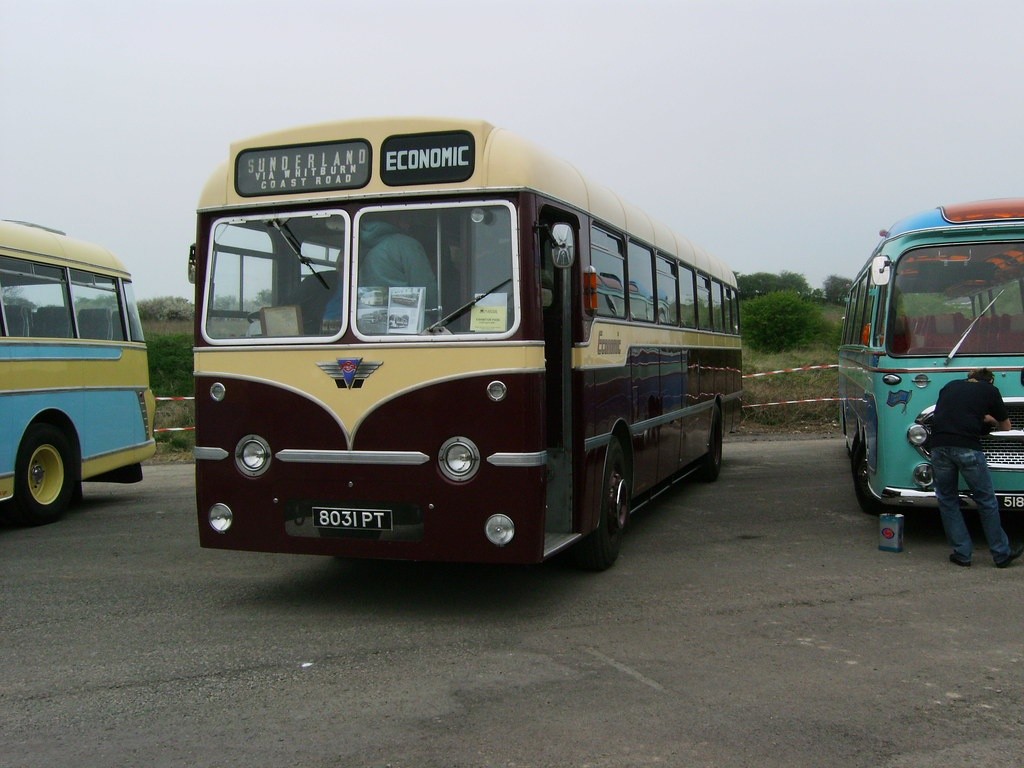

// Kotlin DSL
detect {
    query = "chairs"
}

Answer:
[4,306,141,341]
[892,315,1024,353]
[292,271,338,336]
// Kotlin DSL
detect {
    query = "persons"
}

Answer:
[928,368,1024,568]
[359,211,446,328]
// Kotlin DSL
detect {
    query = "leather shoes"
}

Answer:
[996,544,1024,568]
[950,554,970,566]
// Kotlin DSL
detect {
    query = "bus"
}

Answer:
[188,114,745,576]
[0,218,160,525]
[837,197,1024,516]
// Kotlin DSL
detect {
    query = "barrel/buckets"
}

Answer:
[879,512,904,552]
